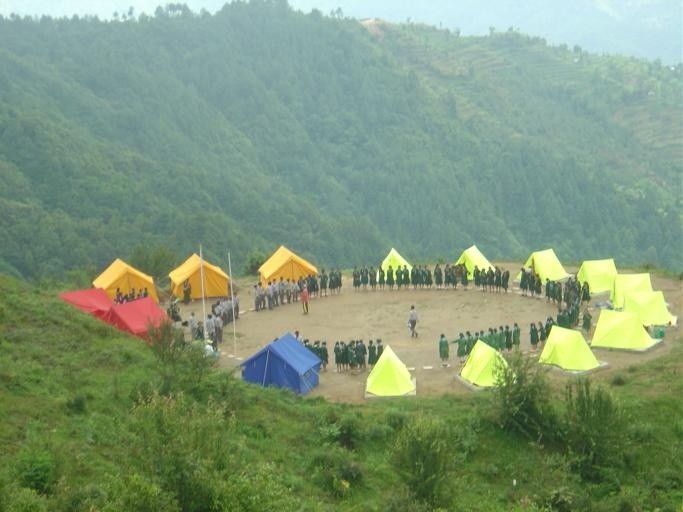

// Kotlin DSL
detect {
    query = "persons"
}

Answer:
[116,261,589,371]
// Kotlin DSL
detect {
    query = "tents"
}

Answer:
[59,287,113,319]
[576,258,674,352]
[94,259,158,304]
[239,332,320,396]
[102,297,170,343]
[167,254,229,300]
[516,246,571,286]
[460,337,520,389]
[373,248,414,283]
[258,246,317,288]
[456,246,497,282]
[365,345,417,398]
[541,324,600,372]
